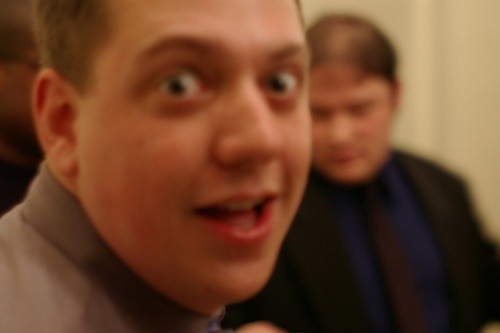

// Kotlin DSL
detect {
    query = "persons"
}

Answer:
[0,0,314,333]
[219,11,500,333]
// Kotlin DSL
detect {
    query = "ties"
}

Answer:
[368,182,429,333]
